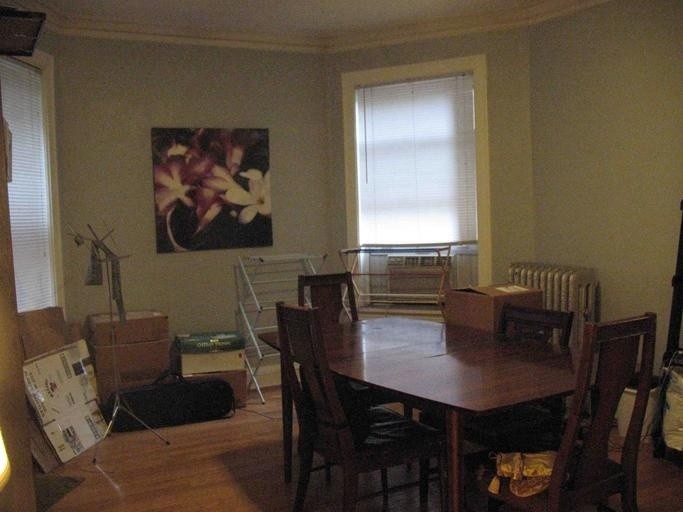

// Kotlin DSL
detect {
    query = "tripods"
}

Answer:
[89,261,169,464]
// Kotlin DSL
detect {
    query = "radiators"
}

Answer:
[509,261,601,359]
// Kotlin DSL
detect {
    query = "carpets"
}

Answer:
[31,471,87,512]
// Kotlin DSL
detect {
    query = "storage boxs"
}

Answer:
[90,342,172,400]
[173,328,245,376]
[179,370,247,409]
[85,309,170,353]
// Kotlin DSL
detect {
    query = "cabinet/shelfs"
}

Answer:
[336,244,454,314]
[232,252,330,404]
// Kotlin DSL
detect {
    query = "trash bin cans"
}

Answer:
[616,373,665,438]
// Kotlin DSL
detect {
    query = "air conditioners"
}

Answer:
[384,252,452,303]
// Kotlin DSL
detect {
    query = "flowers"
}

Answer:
[151,127,272,254]
